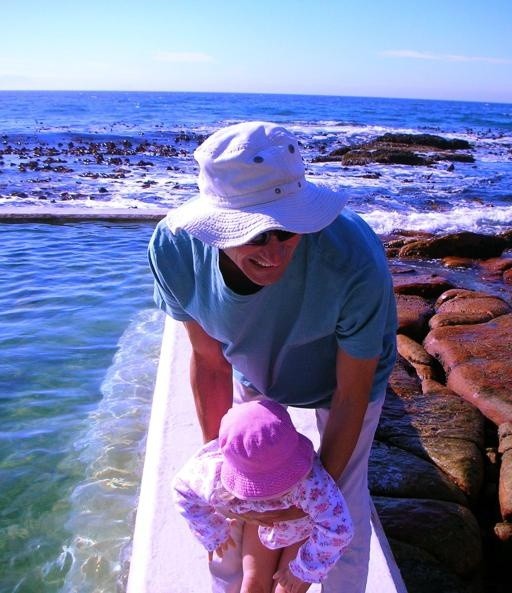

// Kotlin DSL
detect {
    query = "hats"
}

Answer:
[160,118,352,254]
[217,395,315,501]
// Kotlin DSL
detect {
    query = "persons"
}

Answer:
[149,116,400,592]
[169,397,353,592]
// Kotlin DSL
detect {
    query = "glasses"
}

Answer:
[239,228,292,250]
